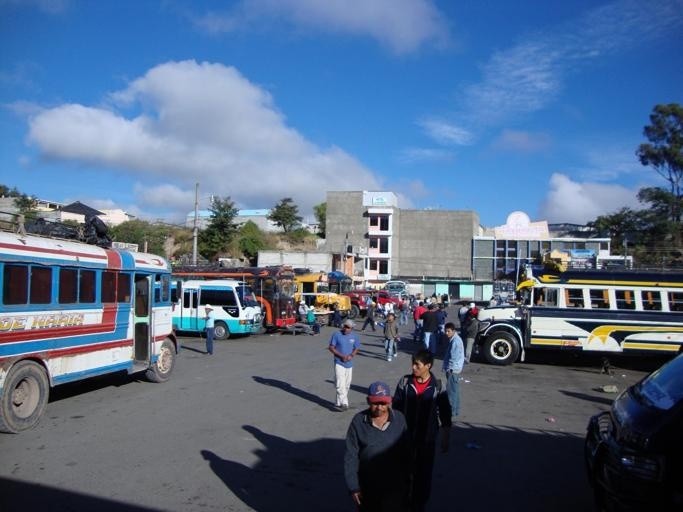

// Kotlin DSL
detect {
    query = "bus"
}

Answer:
[172,265,298,334]
[292,269,353,321]
[155,278,262,341]
[476,261,683,367]
[0,212,180,435]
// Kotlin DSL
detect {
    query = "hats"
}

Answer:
[204,304,213,311]
[368,381,392,405]
[343,319,354,329]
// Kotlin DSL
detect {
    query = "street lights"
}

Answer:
[344,228,354,272]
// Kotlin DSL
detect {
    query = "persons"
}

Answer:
[441,322,465,420]
[201,304,214,356]
[392,349,452,511]
[298,292,499,361]
[329,319,360,412]
[343,380,416,512]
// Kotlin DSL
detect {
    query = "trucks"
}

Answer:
[385,280,408,298]
[373,288,402,312]
[218,256,250,268]
[330,276,374,320]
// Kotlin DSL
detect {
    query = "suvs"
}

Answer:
[583,352,683,511]
